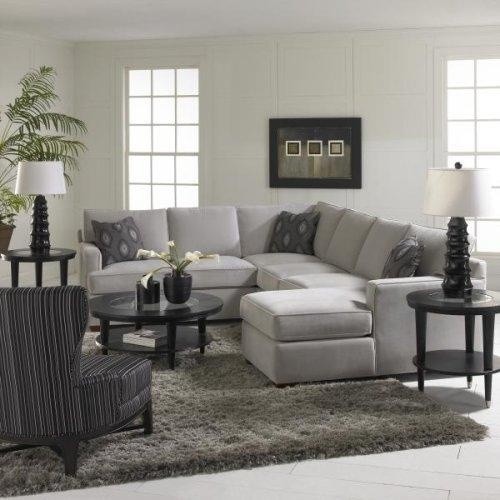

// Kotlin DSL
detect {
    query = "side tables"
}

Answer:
[4,248,77,288]
[406,288,500,409]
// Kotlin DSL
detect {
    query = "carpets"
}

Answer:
[1,324,489,498]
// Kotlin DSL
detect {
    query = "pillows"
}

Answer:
[265,210,321,255]
[381,233,426,279]
[91,216,147,269]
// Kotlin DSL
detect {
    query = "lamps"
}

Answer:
[422,162,489,292]
[15,160,66,249]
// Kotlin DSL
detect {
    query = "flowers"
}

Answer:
[136,239,220,289]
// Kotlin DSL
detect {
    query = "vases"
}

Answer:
[163,272,193,304]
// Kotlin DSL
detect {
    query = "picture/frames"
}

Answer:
[268,116,363,190]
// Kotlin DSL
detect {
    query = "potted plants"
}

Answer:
[0,63,89,258]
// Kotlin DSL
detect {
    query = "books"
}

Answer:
[123,327,168,348]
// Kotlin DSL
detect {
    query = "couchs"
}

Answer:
[75,202,488,387]
[0,285,154,477]
